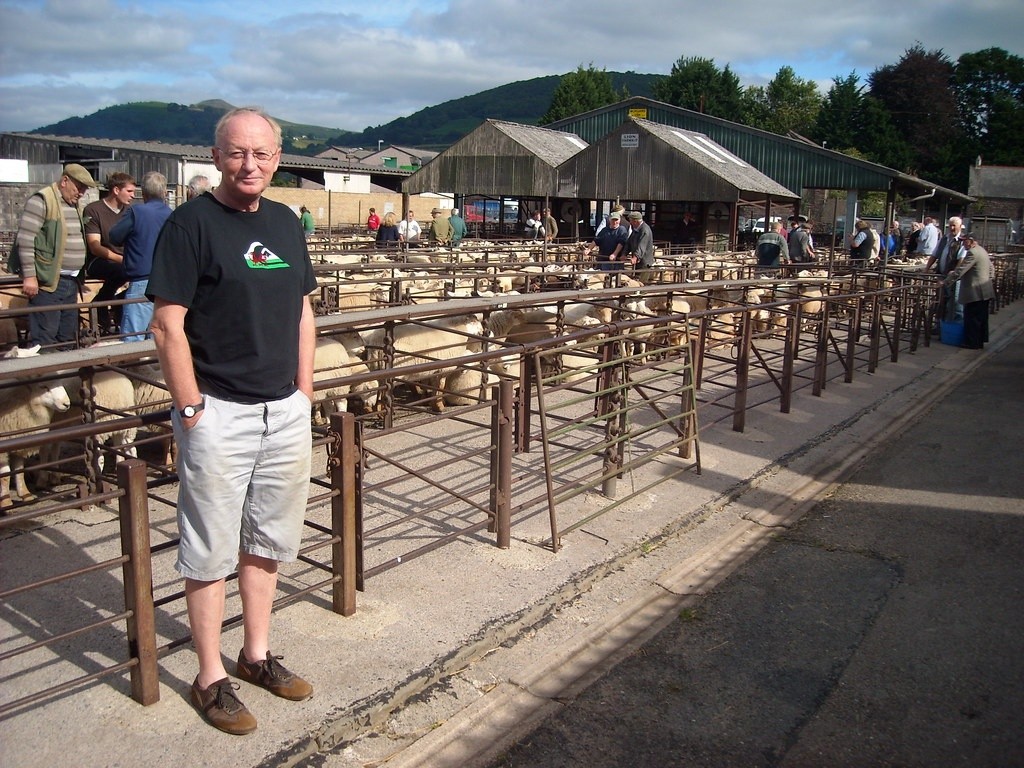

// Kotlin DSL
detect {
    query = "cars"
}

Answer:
[739,215,783,239]
[464,204,498,224]
[832,215,861,236]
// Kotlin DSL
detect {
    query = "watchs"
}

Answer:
[179,403,204,420]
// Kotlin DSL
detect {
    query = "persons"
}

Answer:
[936,232,996,350]
[921,217,968,335]
[493,217,498,223]
[675,211,695,250]
[524,208,558,244]
[399,211,421,247]
[447,208,467,247]
[187,174,211,205]
[429,208,454,246]
[584,205,653,283]
[376,212,403,250]
[16,164,96,356]
[84,171,136,334]
[144,106,319,734]
[847,216,942,268]
[300,207,314,237]
[109,171,174,343]
[755,220,816,278]
[367,208,380,234]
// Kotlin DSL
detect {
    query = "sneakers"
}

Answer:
[191,673,259,735]
[236,646,313,702]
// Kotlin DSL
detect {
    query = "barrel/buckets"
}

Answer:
[940,318,964,345]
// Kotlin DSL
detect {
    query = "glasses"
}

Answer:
[218,147,278,161]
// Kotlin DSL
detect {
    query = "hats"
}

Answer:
[610,212,620,220]
[627,212,642,219]
[64,163,95,190]
[958,232,977,241]
[801,224,811,229]
[431,208,441,214]
[612,205,623,212]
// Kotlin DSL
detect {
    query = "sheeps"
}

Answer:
[346,350,378,408]
[814,269,893,314]
[0,344,70,513]
[686,270,821,348]
[439,240,536,298]
[366,307,525,407]
[522,277,691,360]
[311,327,365,428]
[642,250,757,280]
[86,339,173,433]
[0,280,121,345]
[891,257,937,271]
[4,345,138,481]
[535,242,641,289]
[305,235,438,314]
